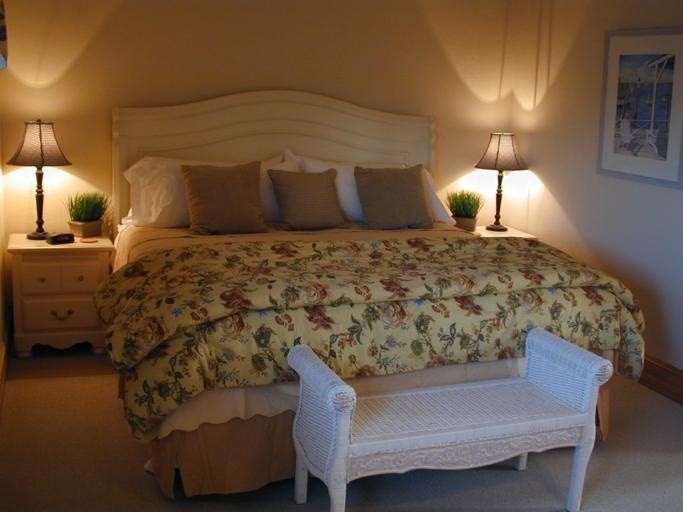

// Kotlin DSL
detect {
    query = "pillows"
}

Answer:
[268,167,352,230]
[284,149,456,226]
[355,164,433,229]
[180,160,268,233]
[123,155,283,228]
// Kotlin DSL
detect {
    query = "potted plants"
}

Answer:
[66,192,111,238]
[446,191,484,231]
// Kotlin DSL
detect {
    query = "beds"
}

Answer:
[112,90,618,499]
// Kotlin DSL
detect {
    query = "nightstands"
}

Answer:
[470,225,536,239]
[6,233,115,357]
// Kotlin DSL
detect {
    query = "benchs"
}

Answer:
[287,327,614,512]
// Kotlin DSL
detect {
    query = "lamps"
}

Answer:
[6,119,72,240]
[474,132,530,232]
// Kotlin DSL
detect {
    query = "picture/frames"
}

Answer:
[596,25,683,190]
